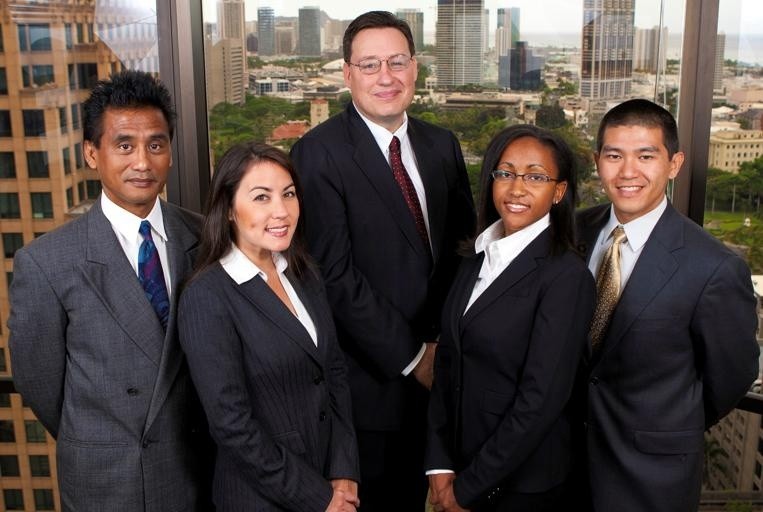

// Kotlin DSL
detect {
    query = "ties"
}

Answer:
[389,136,432,262]
[586,227,627,356]
[138,221,170,335]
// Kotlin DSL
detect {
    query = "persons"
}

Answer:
[286,12,479,512]
[421,122,597,512]
[177,137,364,512]
[576,94,759,511]
[6,71,214,511]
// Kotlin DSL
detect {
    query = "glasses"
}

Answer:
[491,168,559,186]
[347,54,413,75]
